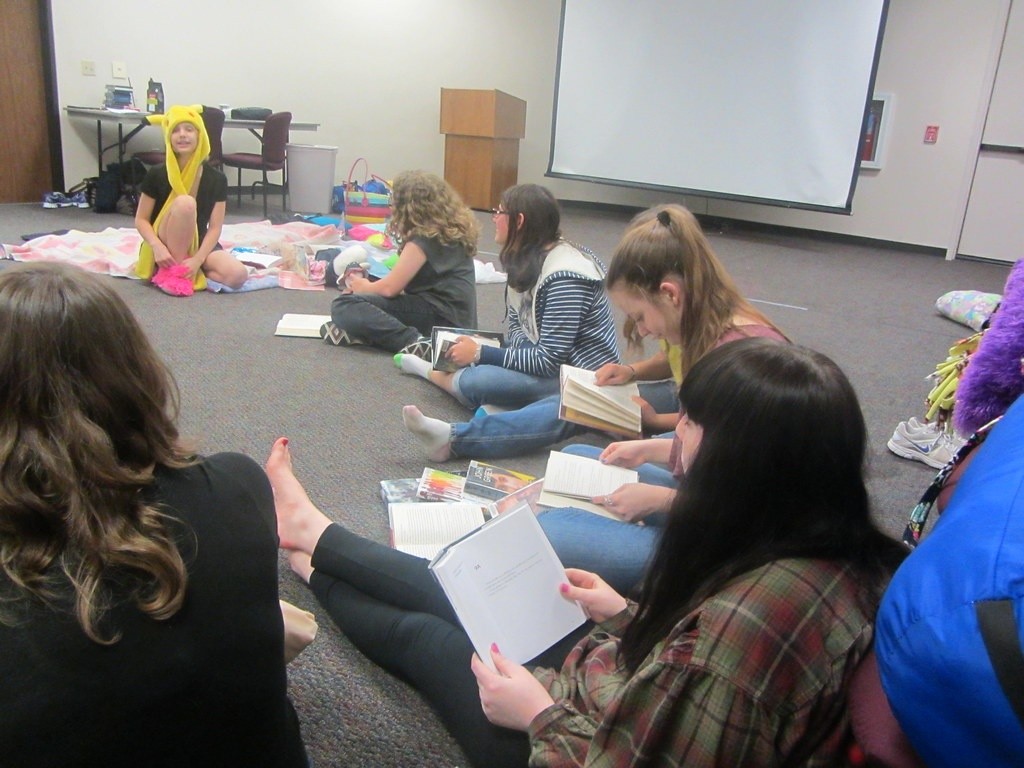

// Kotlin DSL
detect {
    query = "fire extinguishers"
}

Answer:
[862,106,878,161]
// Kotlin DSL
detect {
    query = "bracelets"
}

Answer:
[627,364,634,377]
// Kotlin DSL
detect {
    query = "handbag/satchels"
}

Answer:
[343,158,400,225]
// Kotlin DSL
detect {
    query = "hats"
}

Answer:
[145,105,211,160]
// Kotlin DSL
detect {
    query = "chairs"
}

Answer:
[224,112,292,216]
[131,106,225,172]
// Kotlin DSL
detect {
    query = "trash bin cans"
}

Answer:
[287,143,339,214]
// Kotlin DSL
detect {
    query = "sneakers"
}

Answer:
[71,192,90,209]
[886,417,968,469]
[42,192,73,208]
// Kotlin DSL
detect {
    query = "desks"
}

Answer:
[66,106,320,201]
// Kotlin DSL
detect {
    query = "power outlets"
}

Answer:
[82,59,97,76]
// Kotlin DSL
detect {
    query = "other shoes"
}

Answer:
[320,322,374,346]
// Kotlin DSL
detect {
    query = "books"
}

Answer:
[236,251,282,269]
[536,450,638,521]
[104,84,133,109]
[274,313,332,339]
[427,500,590,674]
[431,326,504,371]
[380,459,544,560]
[559,364,643,440]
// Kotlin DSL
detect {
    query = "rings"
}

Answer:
[604,497,612,505]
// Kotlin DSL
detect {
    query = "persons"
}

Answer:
[0,262,308,768]
[536,205,792,595]
[393,182,622,410]
[264,334,881,768]
[320,170,480,352]
[402,338,683,460]
[469,463,525,494]
[135,104,248,289]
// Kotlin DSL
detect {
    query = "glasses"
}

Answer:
[490,208,510,219]
[387,198,394,209]
